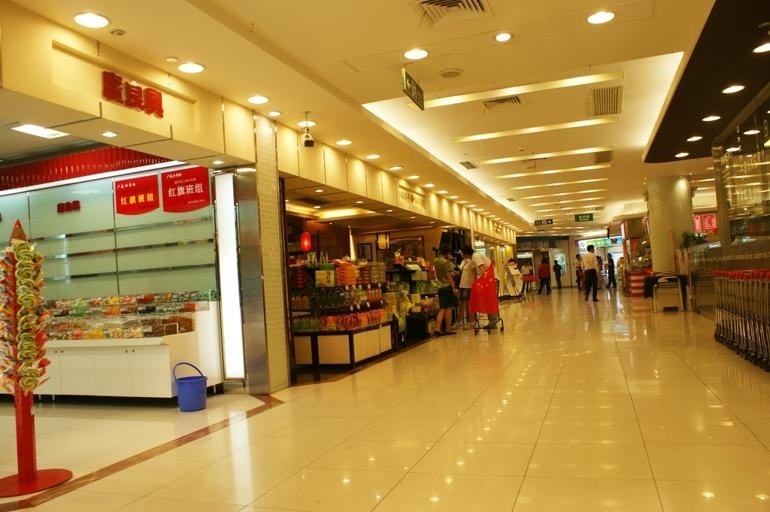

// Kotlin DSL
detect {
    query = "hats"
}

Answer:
[439,243,454,252]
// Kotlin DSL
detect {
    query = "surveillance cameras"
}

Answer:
[301,134,315,149]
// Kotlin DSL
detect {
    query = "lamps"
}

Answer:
[247,95,269,104]
[408,176,420,180]
[54,131,63,135]
[268,111,280,116]
[752,42,770,53]
[178,63,205,74]
[722,85,745,94]
[726,147,739,152]
[10,123,70,139]
[336,140,352,145]
[390,166,402,171]
[297,121,317,127]
[424,183,434,189]
[743,130,760,135]
[687,136,703,142]
[674,152,690,157]
[702,115,721,122]
[587,11,615,24]
[367,154,381,160]
[102,131,118,137]
[73,12,110,28]
[402,49,429,60]
[495,32,513,42]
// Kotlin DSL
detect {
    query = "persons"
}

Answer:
[538,259,550,295]
[606,253,617,289]
[581,245,599,301]
[459,248,476,330]
[394,246,401,258]
[521,262,530,294]
[528,262,533,291]
[596,256,606,289]
[434,244,457,336]
[553,260,562,290]
[462,246,499,329]
[575,254,581,291]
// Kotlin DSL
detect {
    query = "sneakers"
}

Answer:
[434,332,446,337]
[444,330,456,334]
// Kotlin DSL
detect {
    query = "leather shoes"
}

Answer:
[593,299,599,302]
[585,299,588,301]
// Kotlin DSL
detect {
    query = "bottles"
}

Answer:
[288,288,384,311]
[289,308,389,332]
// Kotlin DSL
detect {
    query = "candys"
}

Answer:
[0,241,48,392]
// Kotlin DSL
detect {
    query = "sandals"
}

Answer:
[463,322,475,330]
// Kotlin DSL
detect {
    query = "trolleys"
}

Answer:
[529,279,540,295]
[473,279,504,336]
[713,270,770,373]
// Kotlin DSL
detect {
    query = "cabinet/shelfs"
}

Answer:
[650,272,685,312]
[0,308,94,401]
[291,322,394,368]
[93,301,222,399]
[395,306,456,345]
